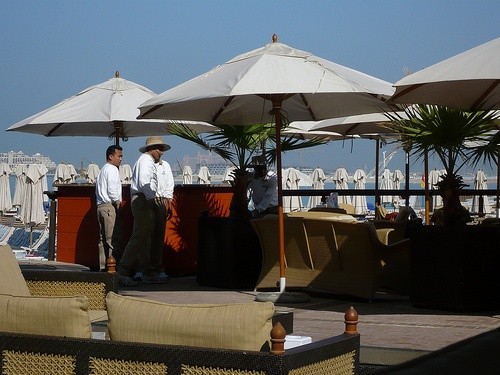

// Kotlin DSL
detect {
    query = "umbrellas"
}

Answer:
[0,159,49,255]
[405,102,500,218]
[382,37,500,112]
[309,103,438,221]
[136,32,415,292]
[204,120,361,155]
[52,158,254,191]
[264,165,492,214]
[3,70,224,145]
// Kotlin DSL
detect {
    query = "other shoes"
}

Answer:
[118,275,138,285]
[144,272,170,283]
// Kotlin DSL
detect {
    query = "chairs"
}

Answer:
[0,216,49,262]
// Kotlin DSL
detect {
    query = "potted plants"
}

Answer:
[375,104,500,312]
[162,108,332,289]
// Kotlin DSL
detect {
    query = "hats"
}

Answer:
[139,136,171,153]
[247,155,270,168]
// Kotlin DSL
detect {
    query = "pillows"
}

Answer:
[0,293,94,339]
[0,244,31,296]
[105,290,275,353]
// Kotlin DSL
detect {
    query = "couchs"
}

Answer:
[0,254,360,375]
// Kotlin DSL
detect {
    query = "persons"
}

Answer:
[95,145,125,273]
[117,136,171,287]
[246,156,281,220]
[134,154,175,282]
